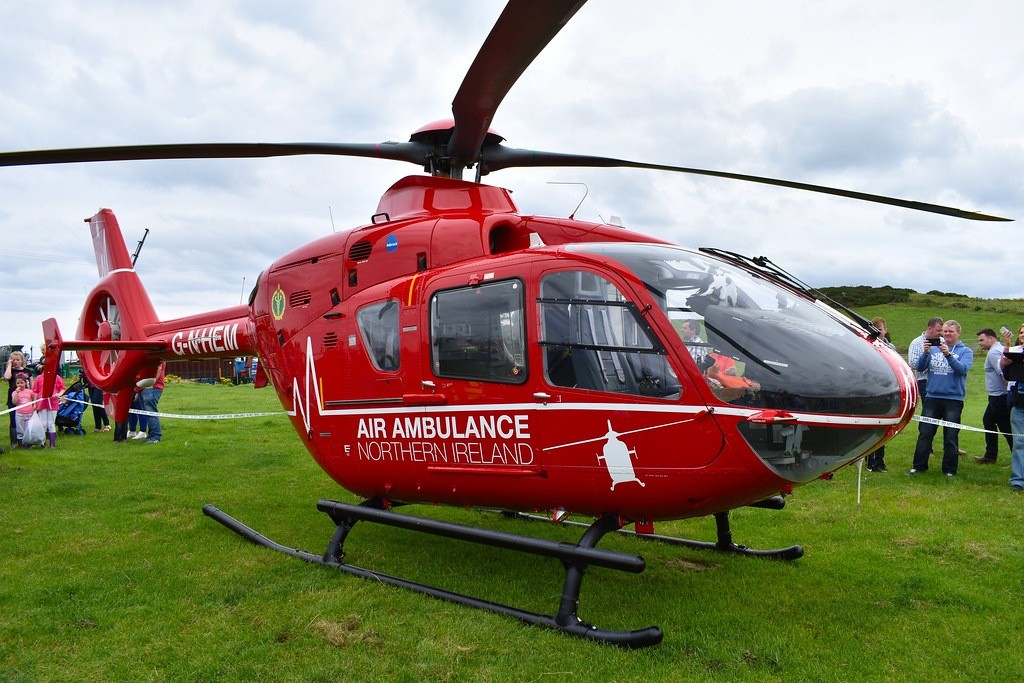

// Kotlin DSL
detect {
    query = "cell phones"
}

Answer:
[926,338,941,346]
[1000,327,1014,339]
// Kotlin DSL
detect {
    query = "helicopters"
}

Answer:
[0,0,1015,650]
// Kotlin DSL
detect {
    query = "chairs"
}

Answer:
[567,297,641,396]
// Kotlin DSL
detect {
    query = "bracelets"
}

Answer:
[1004,347,1009,349]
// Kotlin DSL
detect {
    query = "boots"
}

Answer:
[17,439,23,447]
[41,442,46,448]
[49,433,56,448]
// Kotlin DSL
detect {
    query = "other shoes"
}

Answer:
[127,430,136,438]
[132,431,147,440]
[958,448,967,455]
[904,468,918,477]
[1012,485,1022,492]
[145,438,159,442]
[102,425,112,432]
[866,462,876,472]
[94,429,100,432]
[878,464,888,474]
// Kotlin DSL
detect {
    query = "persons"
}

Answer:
[33,344,66,449]
[904,320,973,477]
[12,377,35,447]
[139,361,166,443]
[867,317,896,473]
[127,393,147,439]
[58,395,68,404]
[4,351,34,449]
[976,321,1024,490]
[908,316,967,457]
[78,371,111,432]
[113,422,128,444]
[681,319,707,375]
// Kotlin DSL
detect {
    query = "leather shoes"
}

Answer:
[974,455,996,464]
